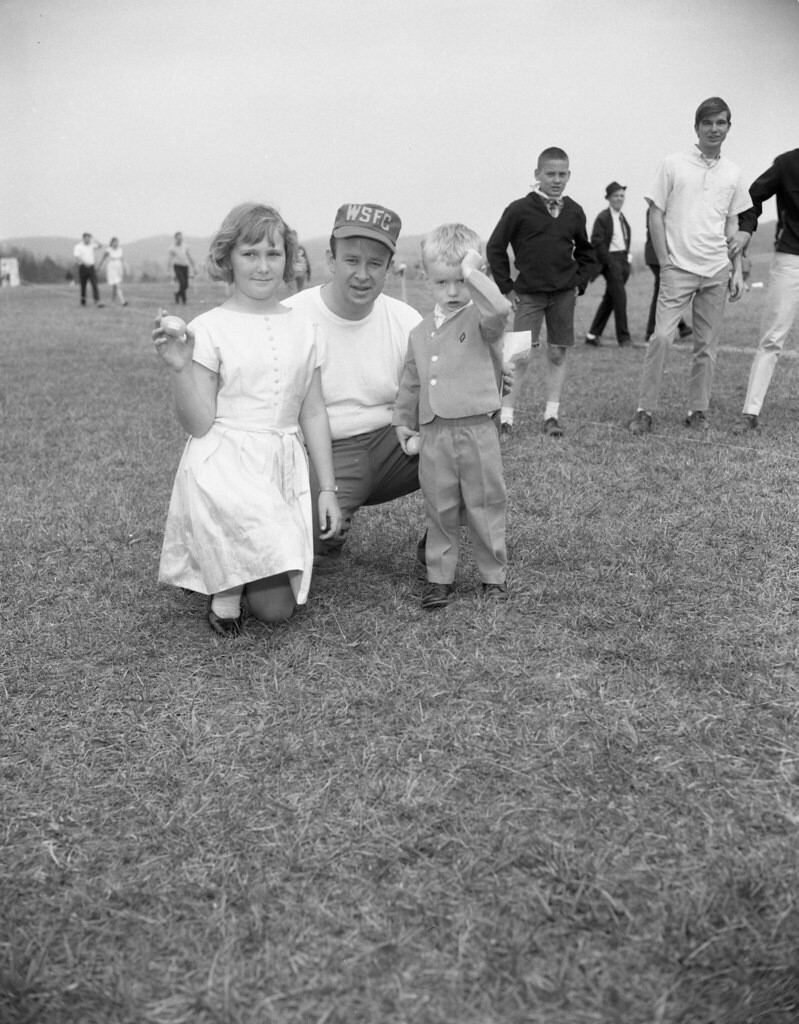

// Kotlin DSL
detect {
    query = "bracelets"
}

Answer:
[318,486,339,495]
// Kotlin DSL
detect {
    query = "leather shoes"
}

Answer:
[206,580,245,637]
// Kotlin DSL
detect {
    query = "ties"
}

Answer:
[619,213,628,253]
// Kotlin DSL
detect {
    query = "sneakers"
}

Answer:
[686,410,710,431]
[628,410,652,437]
[731,412,757,436]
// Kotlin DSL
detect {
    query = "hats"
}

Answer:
[605,181,626,199]
[333,206,403,256]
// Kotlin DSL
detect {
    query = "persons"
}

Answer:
[281,202,516,576]
[165,231,198,305]
[487,147,592,439]
[287,228,312,291]
[73,233,105,308]
[151,201,342,637]
[726,148,799,428]
[584,181,634,348]
[628,96,754,436]
[642,206,694,341]
[390,223,512,609]
[96,237,130,307]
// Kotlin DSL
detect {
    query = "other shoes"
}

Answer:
[679,327,693,337]
[544,417,562,435]
[175,293,179,304]
[95,300,104,307]
[122,300,128,307]
[623,340,634,349]
[421,578,452,606]
[483,582,508,603]
[586,336,602,347]
[312,553,343,574]
[499,423,513,443]
